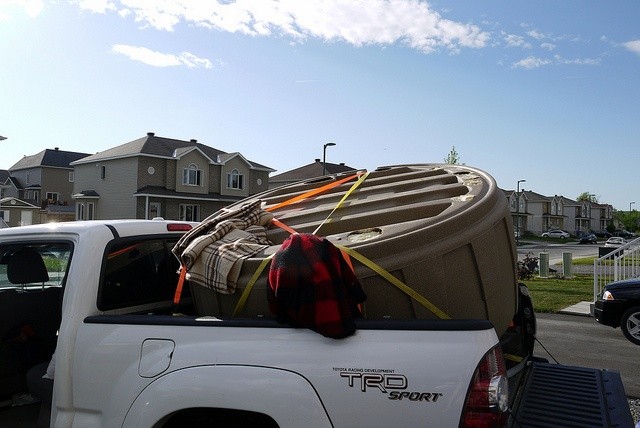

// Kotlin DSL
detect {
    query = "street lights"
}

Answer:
[629,200,635,238]
[320,141,336,180]
[516,179,526,246]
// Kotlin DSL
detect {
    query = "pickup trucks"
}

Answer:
[1,216,635,427]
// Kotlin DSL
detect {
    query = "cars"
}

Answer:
[589,276,640,345]
[541,229,571,240]
[513,226,522,240]
[605,236,631,250]
[597,228,635,238]
[579,232,597,245]
